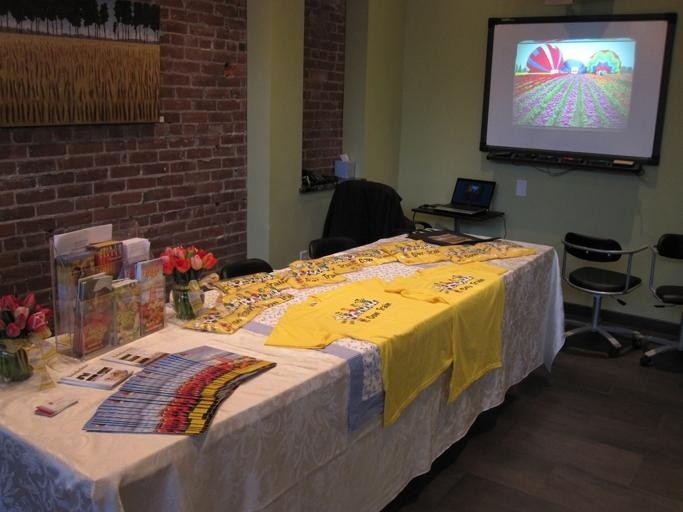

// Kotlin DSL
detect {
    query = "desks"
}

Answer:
[0,226,569,512]
[409,204,506,236]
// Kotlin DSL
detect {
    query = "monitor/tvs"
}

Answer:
[479,13,677,173]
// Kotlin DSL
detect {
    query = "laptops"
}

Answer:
[433,178,496,215]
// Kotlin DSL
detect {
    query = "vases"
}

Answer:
[167,282,207,322]
[0,338,33,383]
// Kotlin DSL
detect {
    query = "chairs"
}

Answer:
[401,210,432,232]
[301,179,402,260]
[556,232,648,366]
[642,234,683,369]
[216,254,273,281]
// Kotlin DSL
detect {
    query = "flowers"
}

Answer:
[155,241,218,316]
[0,290,53,381]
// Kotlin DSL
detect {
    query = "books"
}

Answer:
[56,346,277,434]
[53,224,166,359]
